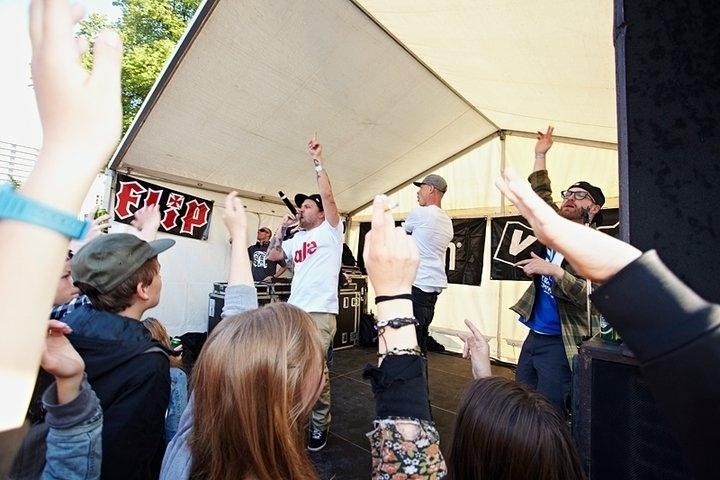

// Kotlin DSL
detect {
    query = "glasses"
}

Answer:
[560,189,596,203]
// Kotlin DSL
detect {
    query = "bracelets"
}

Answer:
[375,293,416,303]
[373,317,419,356]
[273,276,275,279]
[315,165,323,172]
[0,182,90,242]
[535,153,546,159]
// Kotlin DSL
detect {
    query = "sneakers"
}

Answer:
[308,418,329,451]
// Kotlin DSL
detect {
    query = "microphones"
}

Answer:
[277,190,301,220]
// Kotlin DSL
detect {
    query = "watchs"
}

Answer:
[0,182,90,242]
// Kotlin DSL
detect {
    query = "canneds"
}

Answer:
[170,339,182,348]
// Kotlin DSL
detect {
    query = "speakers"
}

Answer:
[614,0,720,302]
[568,339,719,477]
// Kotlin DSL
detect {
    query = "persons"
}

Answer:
[0,0,125,479]
[229,227,288,307]
[142,316,188,445]
[496,166,719,474]
[403,174,453,359]
[160,190,336,477]
[32,233,184,477]
[509,124,606,425]
[363,194,585,479]
[50,251,92,320]
[265,128,345,453]
[40,320,103,477]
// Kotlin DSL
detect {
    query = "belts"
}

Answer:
[529,329,563,340]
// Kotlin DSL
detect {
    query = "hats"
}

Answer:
[69,232,176,297]
[295,193,325,212]
[412,174,448,193]
[569,181,605,208]
